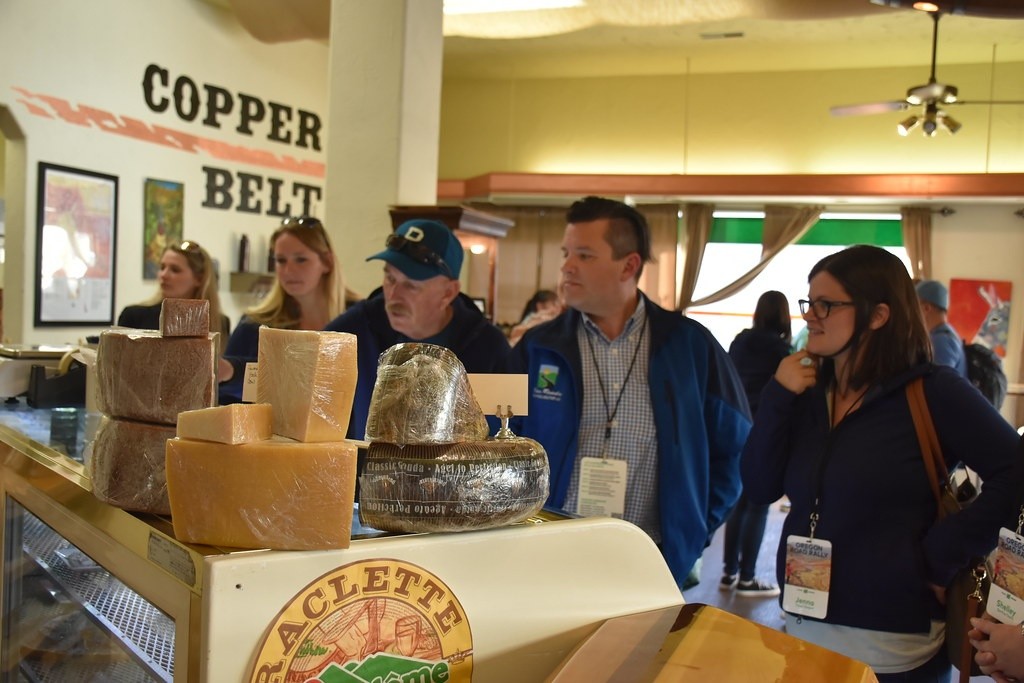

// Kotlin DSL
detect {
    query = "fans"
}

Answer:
[829,0,1024,137]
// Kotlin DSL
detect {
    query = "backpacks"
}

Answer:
[930,329,1006,411]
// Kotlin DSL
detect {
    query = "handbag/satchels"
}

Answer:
[905,379,994,677]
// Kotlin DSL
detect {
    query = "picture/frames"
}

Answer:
[34,162,118,328]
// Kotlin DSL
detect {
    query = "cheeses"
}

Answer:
[85,298,552,553]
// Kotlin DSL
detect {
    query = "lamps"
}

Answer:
[896,0,963,137]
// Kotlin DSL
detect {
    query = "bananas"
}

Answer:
[56,349,81,376]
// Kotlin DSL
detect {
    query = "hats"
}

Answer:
[915,280,948,310]
[366,219,465,280]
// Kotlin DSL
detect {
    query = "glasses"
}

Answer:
[798,300,867,320]
[387,234,456,281]
[281,216,333,254]
[170,239,205,262]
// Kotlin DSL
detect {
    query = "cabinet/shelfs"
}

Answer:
[0,412,689,683]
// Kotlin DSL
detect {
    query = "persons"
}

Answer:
[967,506,1024,683]
[325,219,512,440]
[520,290,563,323]
[911,280,967,379]
[117,241,230,357]
[512,196,754,593]
[740,244,1024,683]
[718,291,796,598]
[218,215,365,406]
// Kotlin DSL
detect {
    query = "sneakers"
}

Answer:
[719,573,740,590]
[736,579,781,596]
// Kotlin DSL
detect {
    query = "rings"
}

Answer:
[800,357,813,365]
